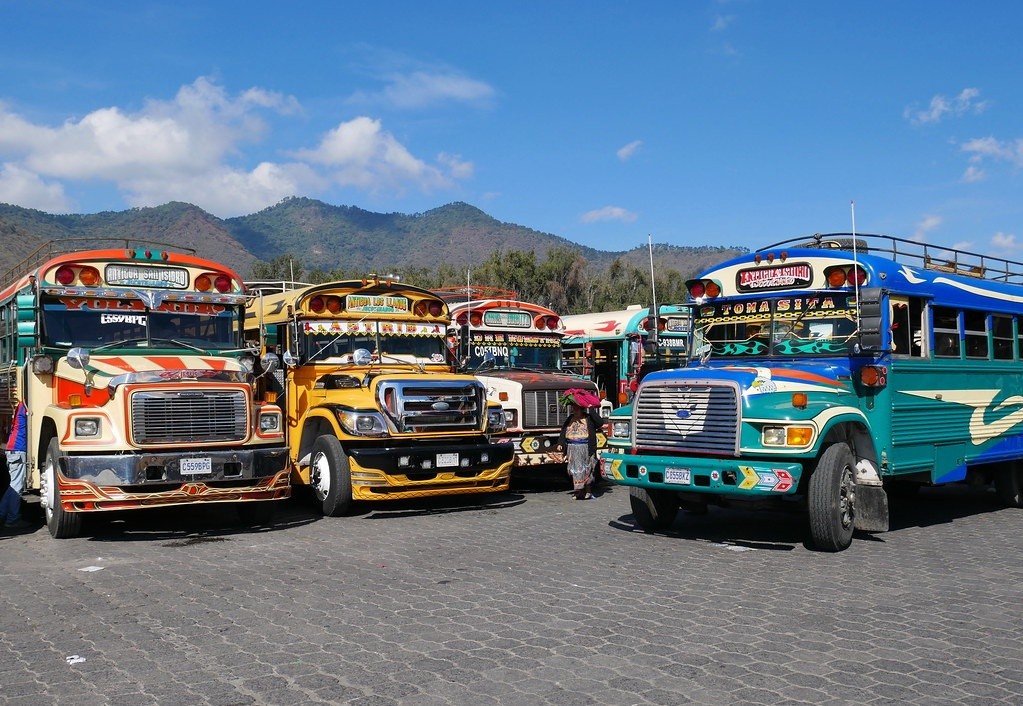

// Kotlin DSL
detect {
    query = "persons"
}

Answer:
[556,402,604,499]
[0,399,28,539]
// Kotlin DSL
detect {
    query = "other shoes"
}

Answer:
[5,520,33,528]
[572,490,594,500]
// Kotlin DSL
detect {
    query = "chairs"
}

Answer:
[152,322,184,347]
[68,321,103,349]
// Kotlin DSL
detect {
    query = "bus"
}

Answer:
[595,197,1023,556]
[553,302,696,415]
[243,256,519,522]
[0,234,294,541]
[424,283,619,482]
